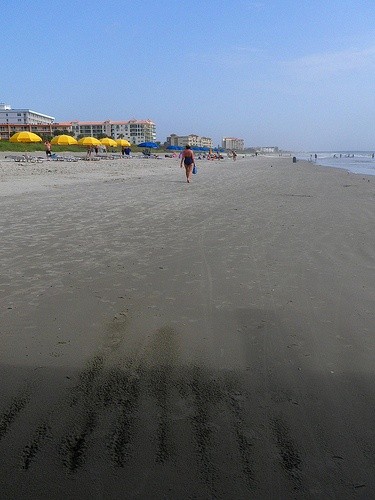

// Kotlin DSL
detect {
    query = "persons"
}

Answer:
[99,144,103,153]
[232,151,236,160]
[142,150,158,157]
[88,146,92,155]
[314,154,317,159]
[94,145,98,156]
[207,153,221,160]
[180,144,195,183]
[44,140,52,159]
[121,147,131,159]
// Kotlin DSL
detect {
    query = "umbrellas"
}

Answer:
[78,136,102,160]
[99,138,118,155]
[51,134,78,154]
[137,142,159,154]
[116,139,131,159]
[166,146,185,151]
[9,130,42,161]
[191,145,224,152]
